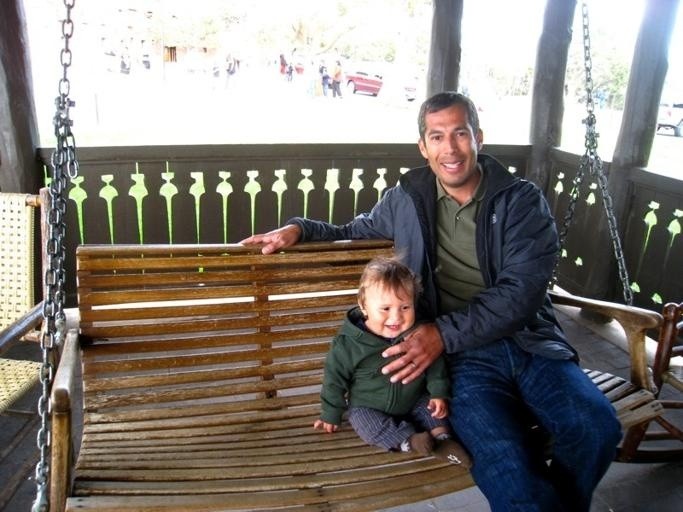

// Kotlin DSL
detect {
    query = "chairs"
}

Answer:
[0,184,55,510]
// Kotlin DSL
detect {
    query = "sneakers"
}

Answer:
[400,431,473,469]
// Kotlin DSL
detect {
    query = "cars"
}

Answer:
[344,60,422,102]
[655,96,683,138]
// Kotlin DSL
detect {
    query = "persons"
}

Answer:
[311,253,451,457]
[318,59,331,96]
[236,90,623,510]
[331,60,344,98]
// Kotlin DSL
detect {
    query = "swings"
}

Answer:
[1,0,663,512]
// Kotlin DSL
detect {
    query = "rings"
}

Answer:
[410,361,418,371]
